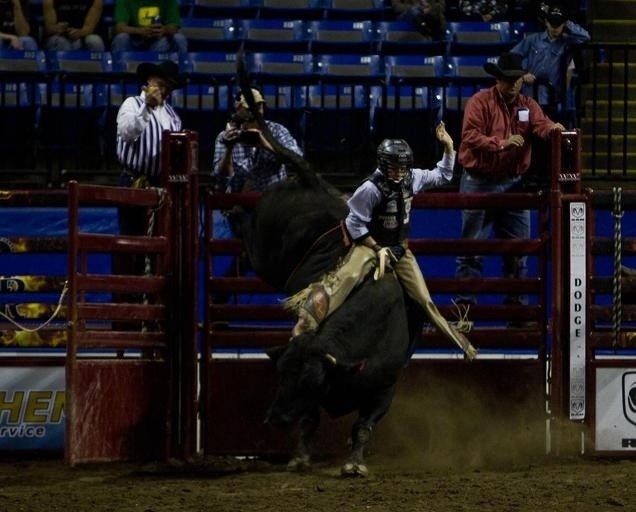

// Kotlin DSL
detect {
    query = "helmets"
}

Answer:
[377,139,414,188]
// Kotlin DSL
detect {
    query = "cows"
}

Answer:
[230,38,411,479]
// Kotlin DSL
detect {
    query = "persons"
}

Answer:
[292,116,479,364]
[510,6,590,135]
[455,52,571,327]
[1,0,38,51]
[392,0,439,36]
[112,0,189,68]
[115,61,174,329]
[210,89,307,216]
[40,0,106,52]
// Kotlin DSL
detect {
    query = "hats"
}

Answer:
[483,53,528,77]
[548,5,568,25]
[137,61,188,89]
[235,88,266,108]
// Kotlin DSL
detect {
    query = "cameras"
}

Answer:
[234,128,261,146]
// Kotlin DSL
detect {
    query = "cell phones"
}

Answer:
[154,16,160,24]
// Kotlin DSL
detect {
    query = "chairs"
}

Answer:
[0,1,525,123]
